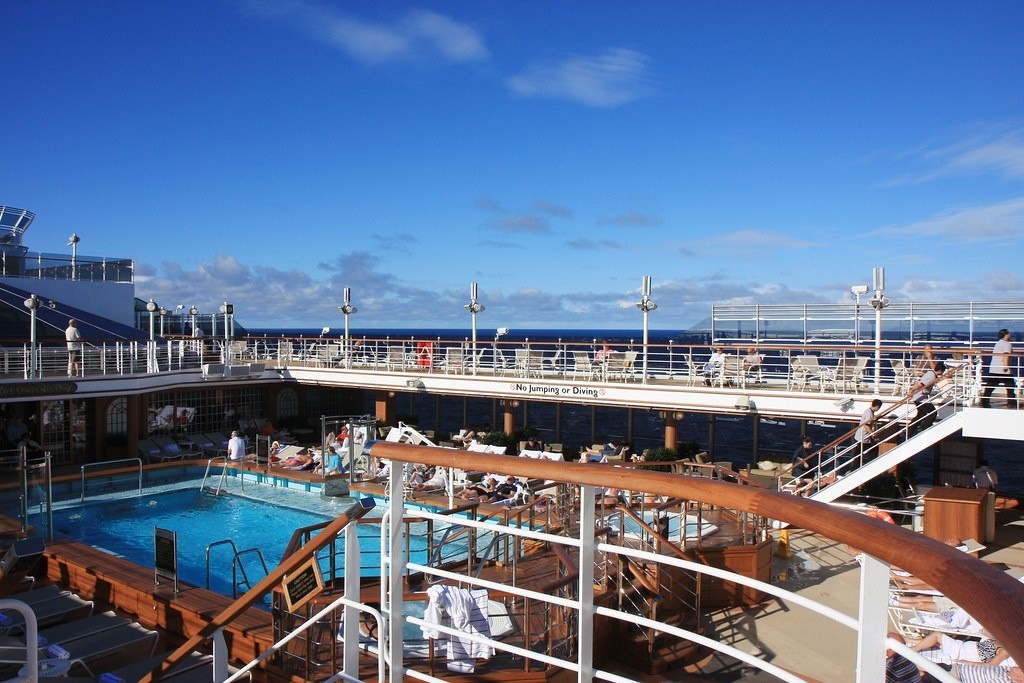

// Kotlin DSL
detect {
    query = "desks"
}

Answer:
[453,480,472,491]
[179,440,194,452]
[817,371,836,394]
[588,365,602,382]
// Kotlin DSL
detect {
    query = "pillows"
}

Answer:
[757,460,777,470]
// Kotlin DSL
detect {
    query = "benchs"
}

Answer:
[854,538,1024,665]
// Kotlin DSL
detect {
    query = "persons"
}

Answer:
[194,322,204,337]
[909,363,946,439]
[453,475,517,504]
[404,463,449,491]
[7,417,44,473]
[333,341,361,366]
[850,399,882,472]
[980,328,1022,408]
[791,435,815,498]
[226,420,385,476]
[701,348,726,386]
[66,319,83,377]
[524,437,541,451]
[585,437,627,456]
[742,347,761,374]
[892,344,939,396]
[885,538,1024,683]
[595,340,613,359]
[458,422,494,442]
[971,459,999,499]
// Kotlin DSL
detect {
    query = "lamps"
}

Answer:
[734,397,755,412]
[407,378,419,388]
[658,411,668,420]
[673,411,684,420]
[500,399,505,406]
[510,400,520,407]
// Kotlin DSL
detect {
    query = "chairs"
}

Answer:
[684,353,766,390]
[137,419,795,511]
[891,357,974,397]
[496,348,563,379]
[789,354,867,394]
[0,584,252,683]
[895,478,925,531]
[573,351,639,383]
[223,339,486,375]
